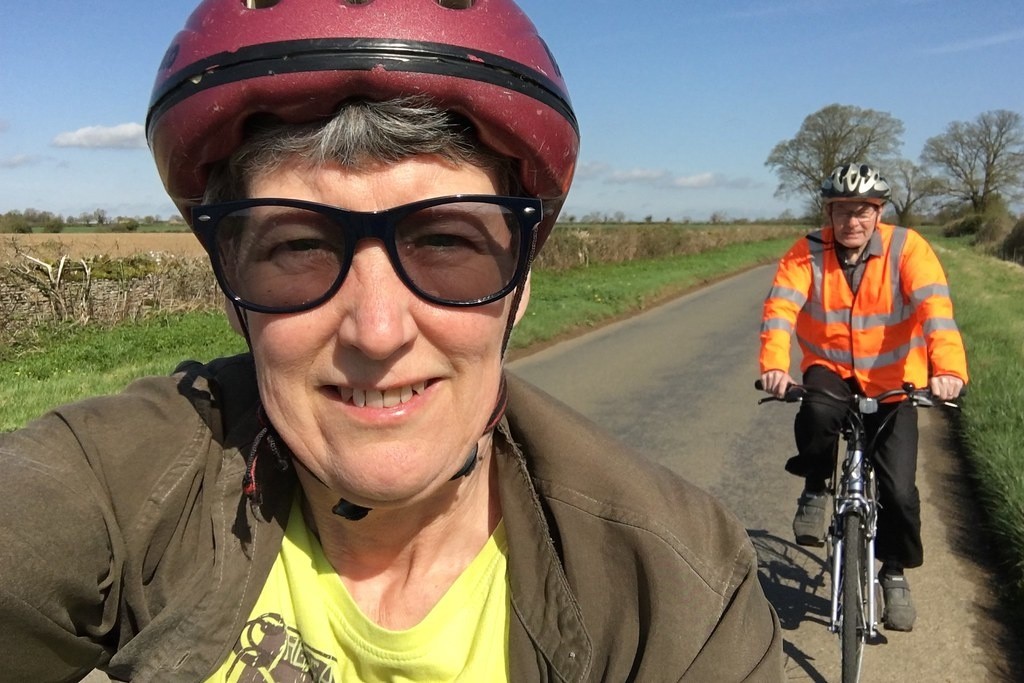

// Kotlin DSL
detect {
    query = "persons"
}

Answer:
[755,157,969,631]
[2,0,788,683]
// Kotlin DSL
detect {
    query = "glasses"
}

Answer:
[191,194,543,314]
[832,207,876,220]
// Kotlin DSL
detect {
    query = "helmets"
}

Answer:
[145,0,582,261]
[820,163,891,206]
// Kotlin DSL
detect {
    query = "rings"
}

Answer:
[947,393,953,396]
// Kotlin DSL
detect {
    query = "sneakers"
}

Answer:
[793,489,827,542]
[877,570,916,630]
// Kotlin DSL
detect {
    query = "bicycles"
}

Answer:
[751,374,961,682]
